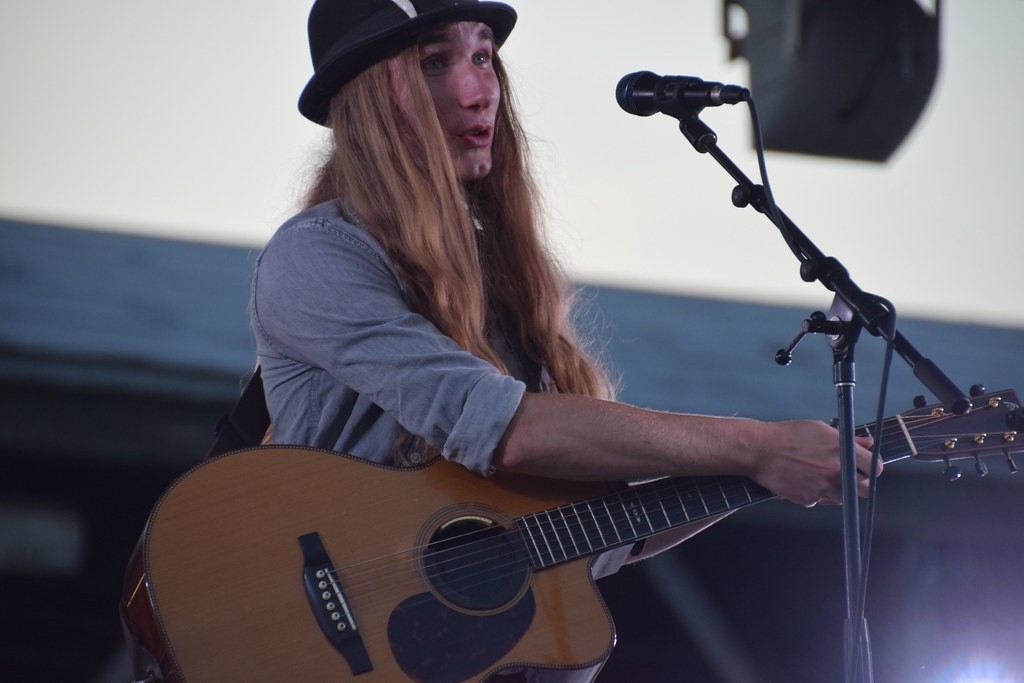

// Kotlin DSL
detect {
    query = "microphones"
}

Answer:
[615,70,747,118]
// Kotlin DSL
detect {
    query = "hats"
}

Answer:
[296,0,517,129]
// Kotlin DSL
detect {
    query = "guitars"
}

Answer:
[122,383,1024,681]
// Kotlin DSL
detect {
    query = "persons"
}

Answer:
[250,0,882,630]
